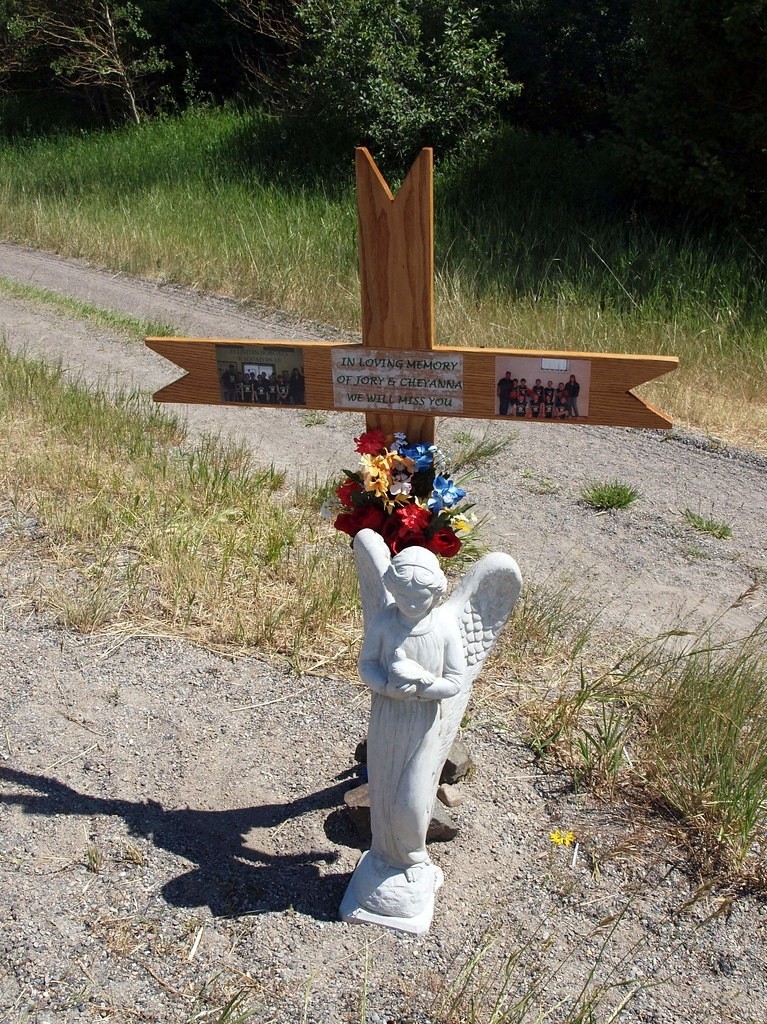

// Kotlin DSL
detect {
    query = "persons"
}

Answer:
[221,364,304,405]
[358,546,464,883]
[497,371,580,419]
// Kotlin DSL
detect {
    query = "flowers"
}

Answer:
[321,430,476,558]
